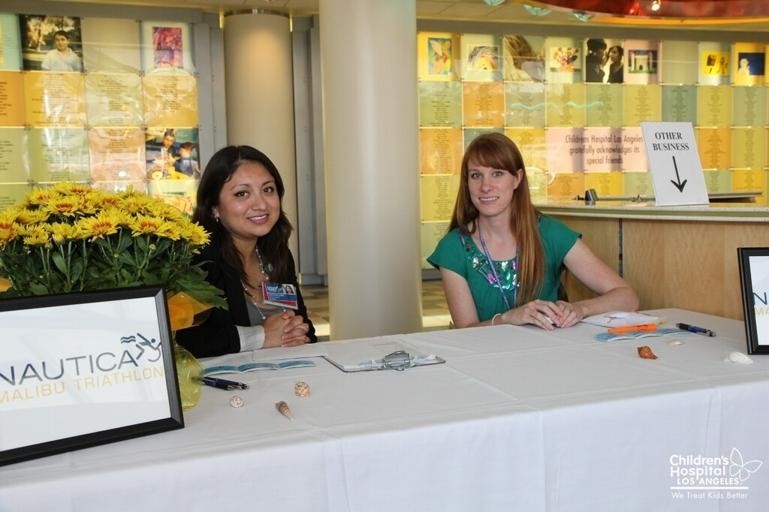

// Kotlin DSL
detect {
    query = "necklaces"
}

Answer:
[239,244,287,323]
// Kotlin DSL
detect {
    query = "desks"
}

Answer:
[0,307,769,512]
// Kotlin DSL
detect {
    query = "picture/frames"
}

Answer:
[0,283,185,468]
[737,246,769,355]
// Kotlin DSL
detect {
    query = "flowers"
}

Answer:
[0,177,230,311]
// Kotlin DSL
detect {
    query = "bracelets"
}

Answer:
[490,312,501,327]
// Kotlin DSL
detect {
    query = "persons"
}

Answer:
[40,29,82,72]
[156,128,179,167]
[284,285,294,294]
[607,46,625,85]
[584,38,606,83]
[426,132,641,332]
[705,53,751,84]
[170,144,318,359]
[172,141,201,179]
[162,29,184,67]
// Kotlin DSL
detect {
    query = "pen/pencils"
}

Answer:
[608,324,656,333]
[192,376,242,391]
[204,377,248,390]
[676,323,716,337]
